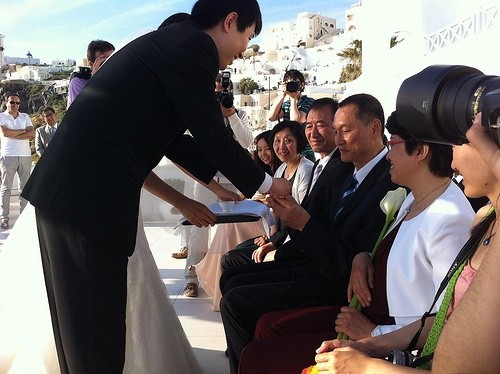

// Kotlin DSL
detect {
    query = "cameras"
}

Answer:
[283,80,300,93]
[216,71,234,109]
[396,59,500,150]
[387,350,413,367]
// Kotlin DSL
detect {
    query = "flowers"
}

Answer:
[336,187,408,340]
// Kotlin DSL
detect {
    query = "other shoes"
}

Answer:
[183,283,198,297]
[2,222,8,229]
[171,249,187,259]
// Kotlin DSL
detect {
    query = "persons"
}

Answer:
[0,94,35,228]
[20,0,290,374]
[171,69,500,374]
[69,40,116,104]
[35,106,59,157]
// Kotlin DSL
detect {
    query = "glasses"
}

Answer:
[9,101,21,105]
[386,140,406,153]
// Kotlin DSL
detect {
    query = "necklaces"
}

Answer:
[483,218,497,246]
[285,159,300,178]
[407,178,450,212]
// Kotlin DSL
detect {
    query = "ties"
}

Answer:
[307,162,323,197]
[49,127,54,134]
[332,173,358,223]
